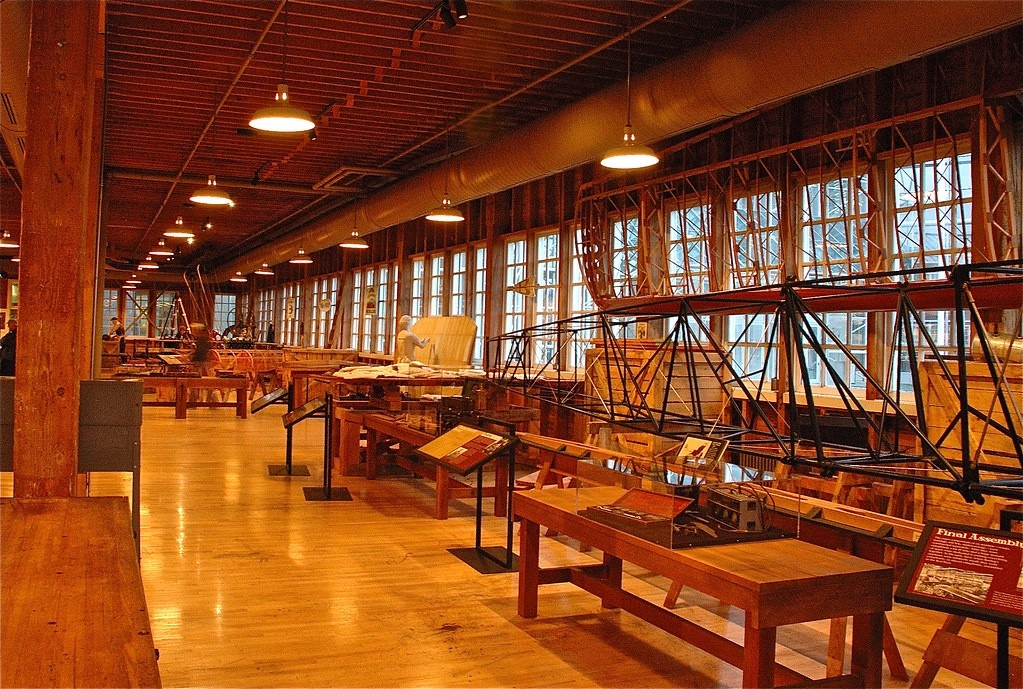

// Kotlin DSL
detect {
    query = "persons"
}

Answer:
[0,319,17,377]
[221,323,274,343]
[108,317,125,364]
[396,315,430,363]
[174,325,189,340]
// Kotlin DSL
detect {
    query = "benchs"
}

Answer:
[250,367,275,400]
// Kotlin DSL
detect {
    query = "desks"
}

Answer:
[0,496,162,689]
[309,374,583,455]
[275,361,379,411]
[363,412,508,518]
[512,487,893,689]
[102,339,277,419]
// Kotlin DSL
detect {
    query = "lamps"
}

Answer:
[0,181,20,247]
[11,254,20,262]
[254,218,274,275]
[230,236,247,282]
[600,0,659,168]
[426,109,464,221]
[338,170,369,248]
[122,87,233,288]
[308,128,317,141]
[288,200,313,263]
[440,0,468,28]
[248,0,315,132]
